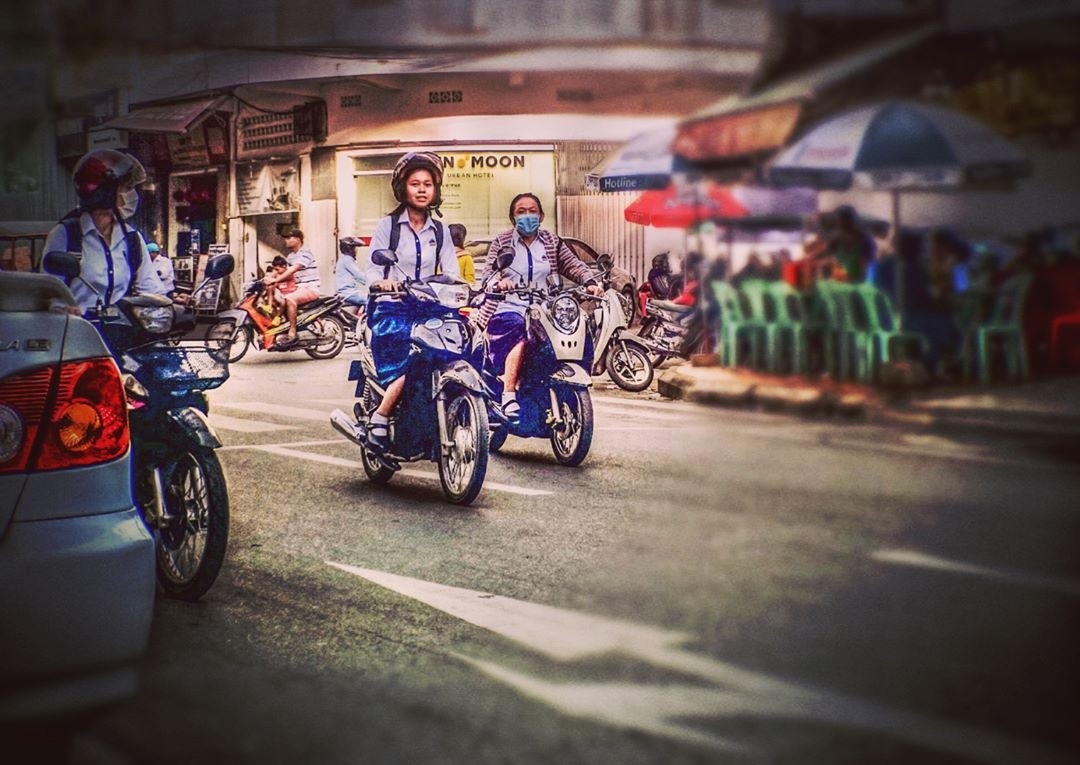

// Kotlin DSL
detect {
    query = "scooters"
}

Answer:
[41,248,236,605]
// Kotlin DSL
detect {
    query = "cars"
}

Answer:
[0,267,155,690]
[455,236,640,329]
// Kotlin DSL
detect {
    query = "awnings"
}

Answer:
[664,17,957,172]
[100,94,231,134]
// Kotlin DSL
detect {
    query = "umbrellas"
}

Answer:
[760,89,1036,231]
[580,129,713,192]
[620,173,820,234]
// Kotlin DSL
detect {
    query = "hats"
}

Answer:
[281,229,304,238]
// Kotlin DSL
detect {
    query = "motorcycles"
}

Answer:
[201,266,345,364]
[333,294,369,350]
[577,255,723,393]
[327,249,514,507]
[483,254,615,470]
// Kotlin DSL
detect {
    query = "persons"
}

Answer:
[146,241,177,297]
[646,200,1079,382]
[334,236,369,305]
[256,228,320,343]
[36,149,192,366]
[365,149,465,451]
[474,193,603,420]
[436,222,474,316]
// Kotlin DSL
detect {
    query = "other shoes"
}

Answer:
[261,303,273,313]
[281,335,298,345]
[366,419,388,450]
[502,401,521,420]
[271,315,281,327]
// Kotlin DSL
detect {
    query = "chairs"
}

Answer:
[710,272,1080,391]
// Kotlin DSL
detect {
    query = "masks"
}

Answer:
[514,214,539,237]
[108,189,139,221]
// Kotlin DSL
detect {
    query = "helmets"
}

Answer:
[340,235,356,253]
[146,242,159,252]
[74,150,132,208]
[392,152,444,203]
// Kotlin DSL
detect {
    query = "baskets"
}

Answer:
[143,339,232,389]
